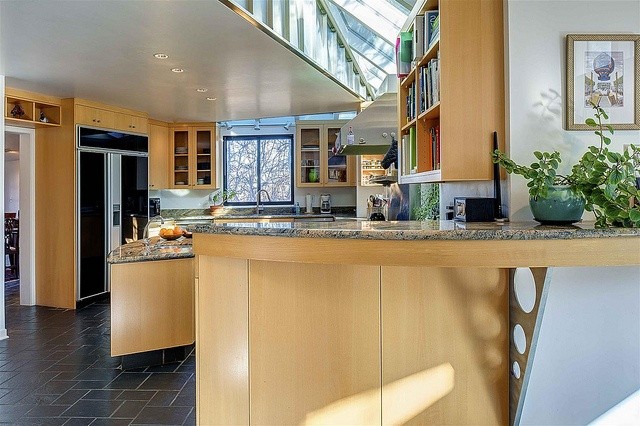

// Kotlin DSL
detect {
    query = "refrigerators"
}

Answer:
[75,123,150,301]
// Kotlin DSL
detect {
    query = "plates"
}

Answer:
[160,234,184,240]
[176,166,188,170]
[176,146,187,154]
[176,182,185,185]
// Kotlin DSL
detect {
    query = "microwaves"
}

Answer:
[454,197,498,222]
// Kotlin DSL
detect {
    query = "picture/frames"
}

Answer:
[567,34,640,131]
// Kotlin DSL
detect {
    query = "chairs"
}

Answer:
[5,218,19,279]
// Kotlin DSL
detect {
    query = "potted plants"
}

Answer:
[489,96,640,231]
[209,188,239,216]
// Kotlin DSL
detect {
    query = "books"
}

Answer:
[406,82,416,123]
[395,32,413,78]
[429,126,440,171]
[420,61,440,114]
[402,126,416,176]
[413,10,440,61]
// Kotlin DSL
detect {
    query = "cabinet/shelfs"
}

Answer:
[171,126,215,189]
[75,104,114,129]
[361,154,389,186]
[115,112,147,133]
[5,96,61,128]
[296,125,350,187]
[395,0,507,184]
[147,123,169,190]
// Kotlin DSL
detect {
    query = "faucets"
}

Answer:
[142,215,164,238]
[256,189,272,216]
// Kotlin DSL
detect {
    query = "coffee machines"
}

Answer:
[320,193,331,213]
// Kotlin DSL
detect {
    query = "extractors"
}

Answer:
[332,93,398,157]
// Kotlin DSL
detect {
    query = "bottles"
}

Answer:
[305,193,313,213]
[295,202,300,215]
